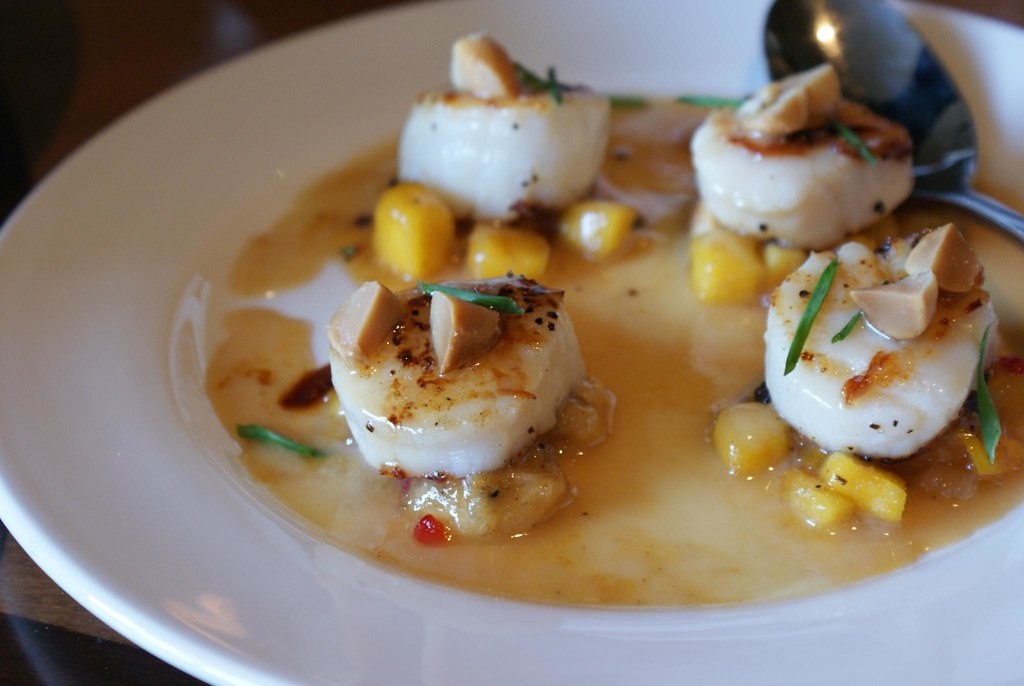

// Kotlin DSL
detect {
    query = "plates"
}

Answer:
[0,0,1024,686]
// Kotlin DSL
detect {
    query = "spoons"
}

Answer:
[761,0,1024,246]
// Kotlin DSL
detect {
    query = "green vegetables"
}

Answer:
[236,56,1000,459]
